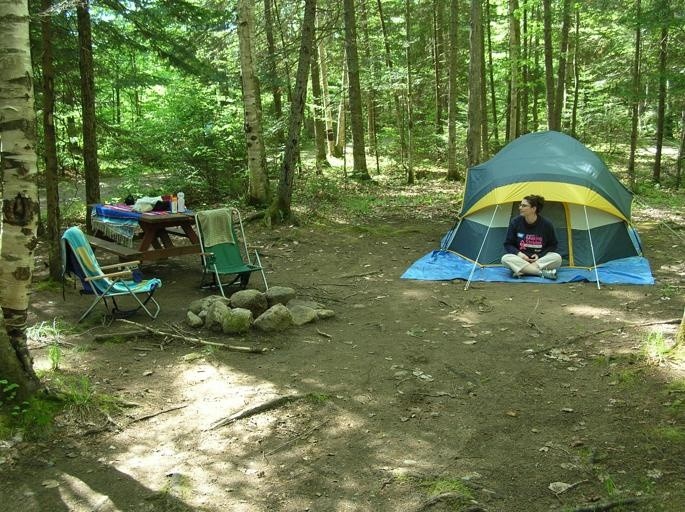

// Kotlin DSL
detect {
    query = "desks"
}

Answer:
[84,203,204,271]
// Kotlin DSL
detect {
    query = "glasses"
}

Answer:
[518,204,528,208]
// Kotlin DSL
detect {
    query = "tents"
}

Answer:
[441,131,644,270]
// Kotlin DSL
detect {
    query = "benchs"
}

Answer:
[165,227,187,237]
[83,233,142,270]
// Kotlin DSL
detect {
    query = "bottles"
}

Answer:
[162,191,185,213]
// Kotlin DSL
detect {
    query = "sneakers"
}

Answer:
[543,268,557,279]
[513,272,524,277]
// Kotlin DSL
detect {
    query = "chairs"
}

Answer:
[195,207,268,297]
[61,226,162,323]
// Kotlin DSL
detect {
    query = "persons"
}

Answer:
[501,196,566,278]
